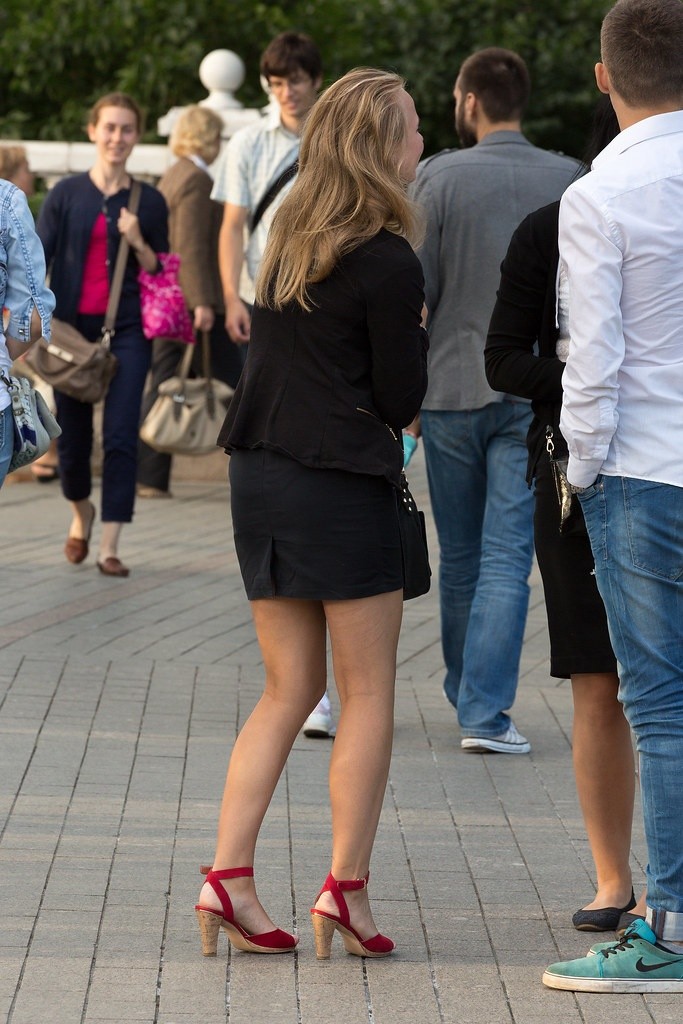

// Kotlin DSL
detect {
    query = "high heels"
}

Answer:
[311,871,393,960]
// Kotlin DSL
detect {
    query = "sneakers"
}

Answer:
[460,722,531,754]
[194,866,297,955]
[542,918,683,993]
[304,694,337,737]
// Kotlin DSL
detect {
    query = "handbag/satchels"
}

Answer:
[7,376,62,473]
[138,327,235,456]
[22,318,118,403]
[553,459,585,535]
[137,253,198,345]
[397,481,432,601]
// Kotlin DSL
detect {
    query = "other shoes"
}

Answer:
[135,484,173,499]
[98,557,128,577]
[571,886,637,932]
[32,462,57,482]
[617,912,646,936]
[66,502,96,565]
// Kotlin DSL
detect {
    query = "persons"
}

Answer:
[542,2,682,993]
[483,92,634,922]
[189,65,424,957]
[209,33,325,366]
[406,48,584,752]
[0,178,56,488]
[0,145,57,484]
[138,102,225,499]
[37,94,171,582]
